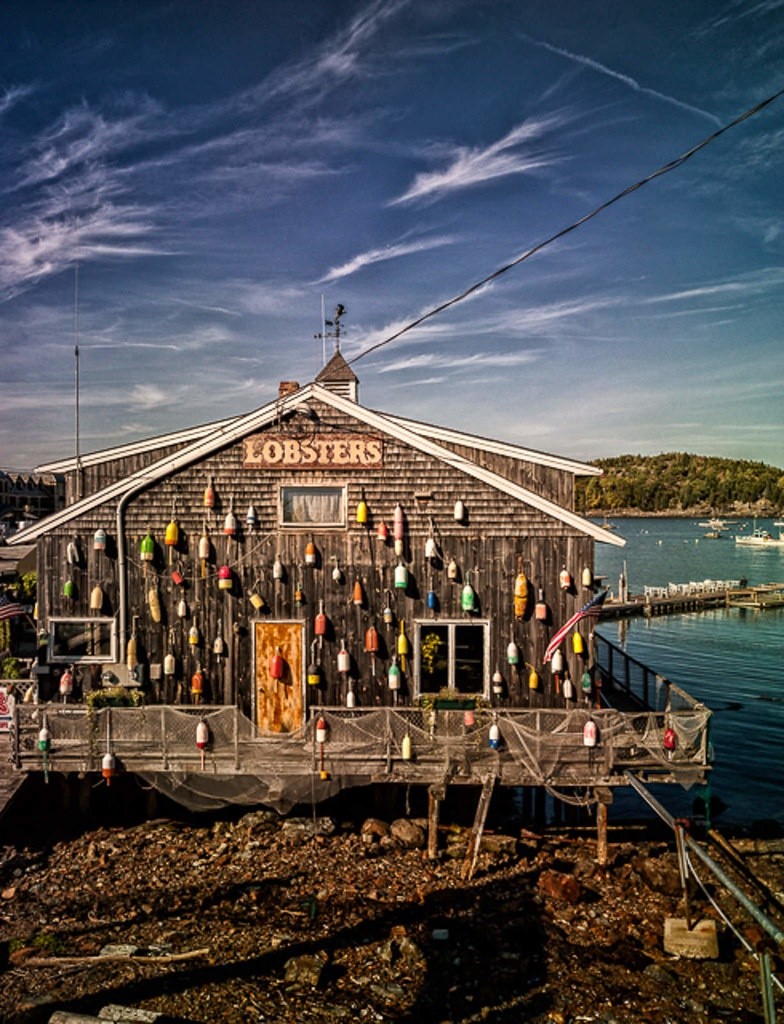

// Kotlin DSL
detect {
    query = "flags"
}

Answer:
[542,591,608,665]
[0,594,26,621]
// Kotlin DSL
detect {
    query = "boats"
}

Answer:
[704,531,720,539]
[701,516,731,531]
[736,520,782,547]
[601,517,617,531]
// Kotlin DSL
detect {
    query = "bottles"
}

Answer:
[36,478,676,779]
